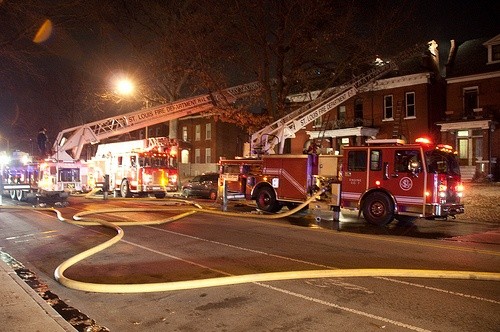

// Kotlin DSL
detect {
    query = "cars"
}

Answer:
[182,172,219,200]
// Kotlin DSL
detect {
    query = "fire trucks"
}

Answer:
[78,136,178,199]
[0,78,274,202]
[219,43,465,229]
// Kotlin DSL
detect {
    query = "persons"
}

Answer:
[37,127,48,157]
